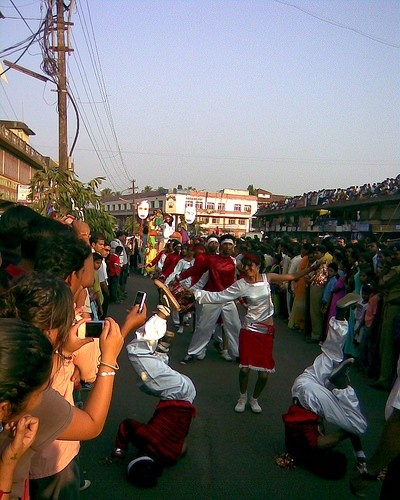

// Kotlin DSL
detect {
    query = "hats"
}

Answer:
[313,245,327,253]
[389,240,400,251]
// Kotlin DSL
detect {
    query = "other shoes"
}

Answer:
[197,351,206,360]
[307,339,320,343]
[187,313,191,318]
[80,384,93,390]
[221,349,232,360]
[368,383,387,390]
[184,320,190,326]
[177,326,184,333]
[121,292,130,300]
[79,479,91,492]
[174,324,180,327]
[319,341,324,346]
[213,340,221,354]
[114,300,124,303]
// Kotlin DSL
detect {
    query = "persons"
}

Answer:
[109,278,198,489]
[281,291,370,481]
[179,232,249,366]
[185,248,327,414]
[0,317,55,500]
[0,201,400,500]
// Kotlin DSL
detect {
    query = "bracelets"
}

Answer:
[97,364,117,372]
[115,447,124,455]
[351,449,366,458]
[55,349,73,362]
[0,490,12,500]
[96,372,116,377]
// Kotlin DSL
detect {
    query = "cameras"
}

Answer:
[85,321,106,338]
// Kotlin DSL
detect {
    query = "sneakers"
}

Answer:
[180,354,193,365]
[159,331,175,344]
[249,399,262,412]
[327,358,354,385]
[235,395,247,412]
[335,293,359,311]
[154,279,180,315]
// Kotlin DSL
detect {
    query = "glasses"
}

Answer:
[104,248,111,252]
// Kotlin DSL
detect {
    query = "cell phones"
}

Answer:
[133,291,146,314]
